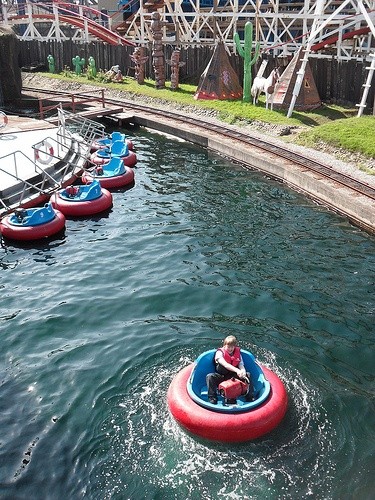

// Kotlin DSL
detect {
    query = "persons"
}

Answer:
[207,335,256,404]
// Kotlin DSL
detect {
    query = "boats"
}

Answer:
[90,139,138,168]
[50,178,113,217]
[81,157,135,189]
[167,347,289,443]
[92,130,133,151]
[0,200,66,241]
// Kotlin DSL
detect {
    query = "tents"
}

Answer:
[264,46,321,110]
[194,40,243,100]
